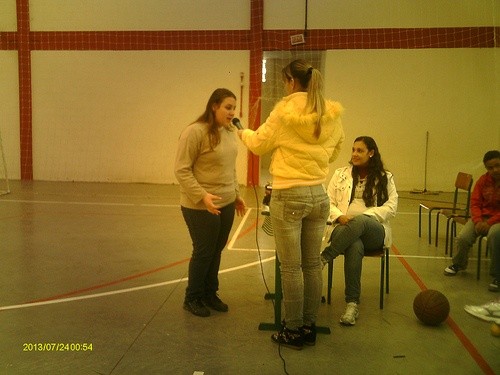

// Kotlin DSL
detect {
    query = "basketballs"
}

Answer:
[413,289,450,326]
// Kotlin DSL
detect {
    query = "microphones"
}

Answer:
[232,118,243,130]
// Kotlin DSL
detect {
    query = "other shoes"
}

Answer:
[488,278,500,289]
[444,263,467,274]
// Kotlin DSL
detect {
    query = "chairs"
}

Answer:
[327,245,390,309]
[418,172,473,245]
[435,209,471,254]
[450,216,471,257]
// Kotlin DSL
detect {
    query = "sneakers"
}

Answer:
[302,323,316,346]
[340,301,360,326]
[463,301,500,325]
[270,325,305,349]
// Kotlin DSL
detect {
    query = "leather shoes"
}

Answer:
[199,290,228,312]
[183,294,210,317]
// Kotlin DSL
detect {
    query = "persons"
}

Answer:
[442,150,500,293]
[465,298,500,325]
[315,137,398,326]
[174,88,246,318]
[238,58,345,352]
